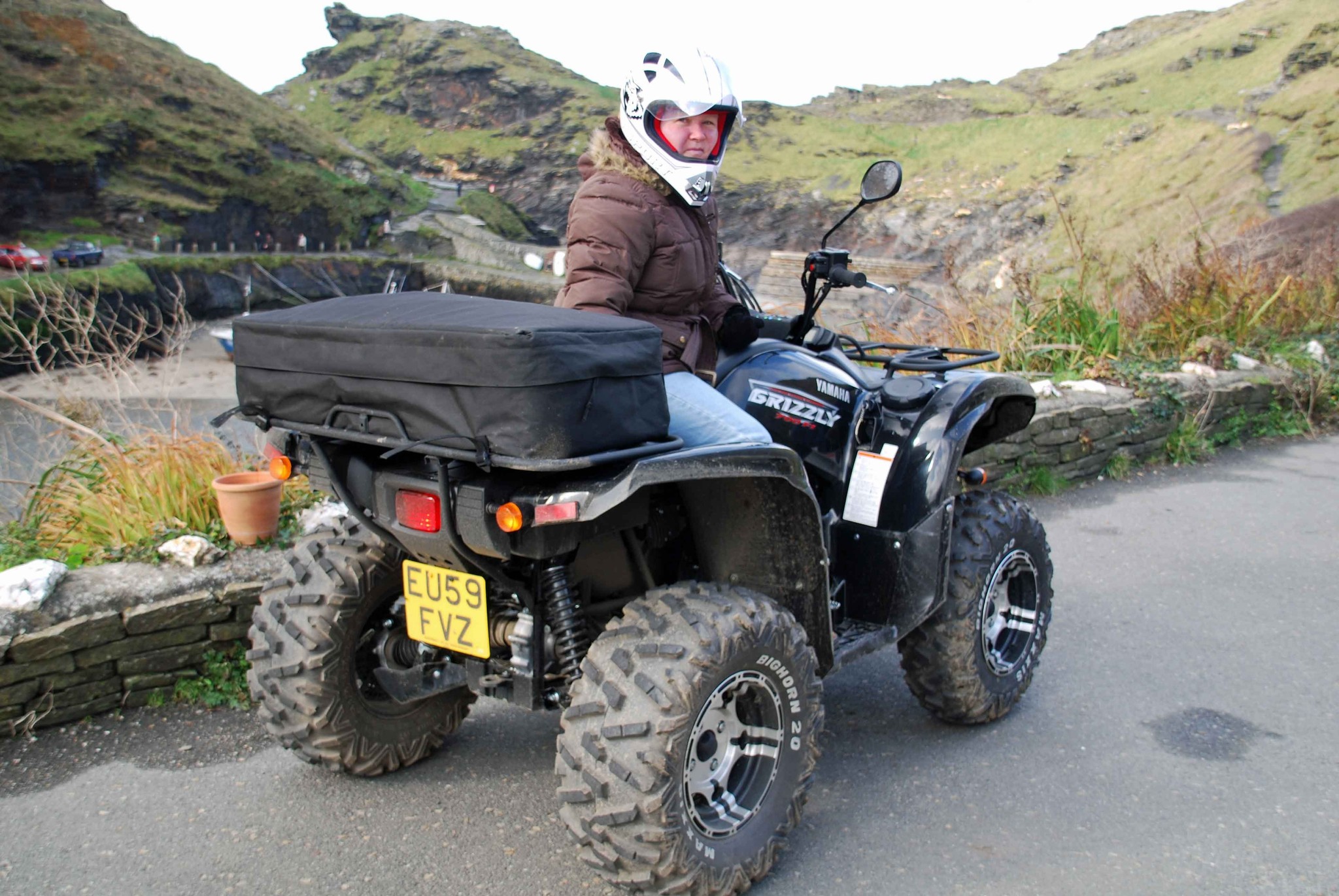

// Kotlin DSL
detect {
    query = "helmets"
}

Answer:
[619,44,744,207]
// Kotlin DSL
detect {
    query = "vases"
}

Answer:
[213,475,286,542]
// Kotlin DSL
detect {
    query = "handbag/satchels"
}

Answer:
[231,290,671,463]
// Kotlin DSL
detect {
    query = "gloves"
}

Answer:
[718,304,766,355]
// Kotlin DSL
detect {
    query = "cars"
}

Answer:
[0,244,49,271]
[53,241,103,268]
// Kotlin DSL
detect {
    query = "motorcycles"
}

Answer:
[245,158,1055,896]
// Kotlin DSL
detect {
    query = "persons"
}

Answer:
[551,44,837,643]
[151,232,161,252]
[456,180,462,197]
[298,233,307,253]
[377,220,391,247]
[18,240,26,249]
[255,231,274,254]
